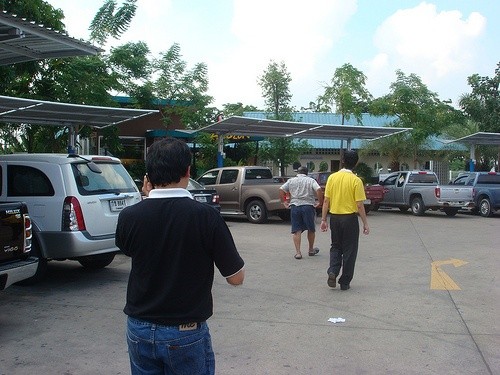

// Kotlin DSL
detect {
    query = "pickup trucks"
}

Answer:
[372,171,475,217]
[307,171,384,217]
[0,200,40,290]
[195,165,292,224]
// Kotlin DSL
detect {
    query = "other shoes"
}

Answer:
[327,271,336,287]
[308,247,319,255]
[295,252,302,258]
[340,284,350,290]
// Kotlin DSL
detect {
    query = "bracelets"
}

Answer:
[320,218,327,221]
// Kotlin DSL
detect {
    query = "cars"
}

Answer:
[134,177,222,213]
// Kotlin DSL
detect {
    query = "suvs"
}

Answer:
[445,172,500,217]
[0,150,142,288]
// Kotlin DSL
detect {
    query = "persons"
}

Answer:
[279,167,322,259]
[320,150,369,289]
[115,137,244,375]
